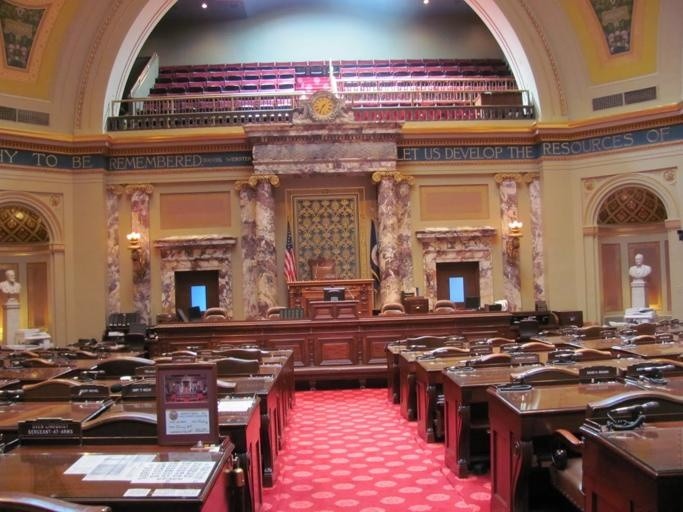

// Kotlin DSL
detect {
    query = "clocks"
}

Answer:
[307,91,343,124]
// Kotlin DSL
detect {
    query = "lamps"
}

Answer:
[126,231,141,249]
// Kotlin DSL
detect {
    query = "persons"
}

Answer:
[0,270,20,304]
[629,254,651,283]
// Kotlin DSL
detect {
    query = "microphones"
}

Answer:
[612,401,660,415]
[111,383,134,394]
[637,364,675,372]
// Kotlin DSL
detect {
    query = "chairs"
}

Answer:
[201,300,457,320]
[145,58,512,113]
[385,325,681,509]
[2,345,299,512]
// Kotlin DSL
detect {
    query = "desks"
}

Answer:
[147,312,519,393]
